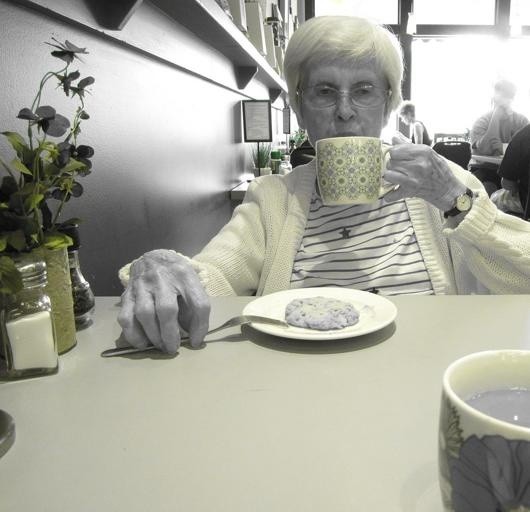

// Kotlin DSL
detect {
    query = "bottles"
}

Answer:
[39,223,94,330]
[278,155,292,174]
[22,248,76,355]
[0,258,59,379]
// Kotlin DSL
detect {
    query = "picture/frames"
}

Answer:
[240,100,273,143]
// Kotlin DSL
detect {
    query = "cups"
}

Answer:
[316,136,397,207]
[437,349,530,511]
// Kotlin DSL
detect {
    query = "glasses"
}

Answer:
[296,85,392,108]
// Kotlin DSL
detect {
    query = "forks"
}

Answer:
[101,315,288,357]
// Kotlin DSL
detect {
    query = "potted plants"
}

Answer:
[0,36,95,322]
[252,142,272,177]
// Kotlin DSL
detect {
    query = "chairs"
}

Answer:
[432,141,472,171]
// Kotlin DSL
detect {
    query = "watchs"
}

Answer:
[443,187,473,218]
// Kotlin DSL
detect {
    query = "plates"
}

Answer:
[241,286,397,341]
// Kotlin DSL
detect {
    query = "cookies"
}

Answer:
[286,296,359,331]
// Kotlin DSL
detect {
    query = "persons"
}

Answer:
[117,16,530,355]
[468,80,529,195]
[499,123,530,222]
[391,103,431,146]
